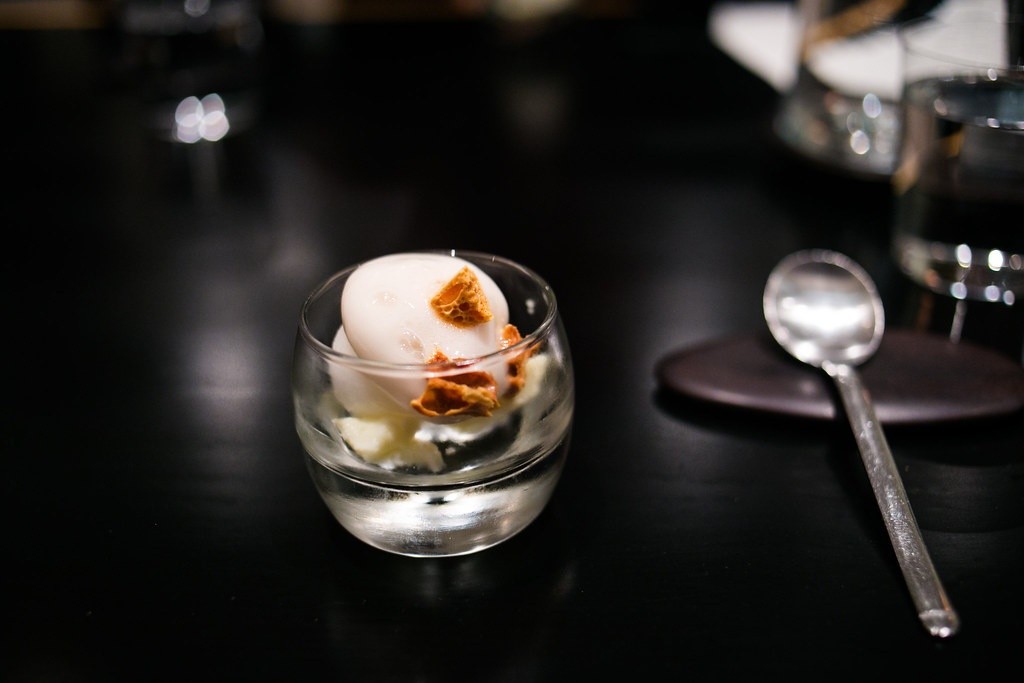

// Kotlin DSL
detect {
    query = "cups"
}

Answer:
[889,14,1024,305]
[289,250,574,558]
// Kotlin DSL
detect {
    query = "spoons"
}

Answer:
[762,249,960,638]
